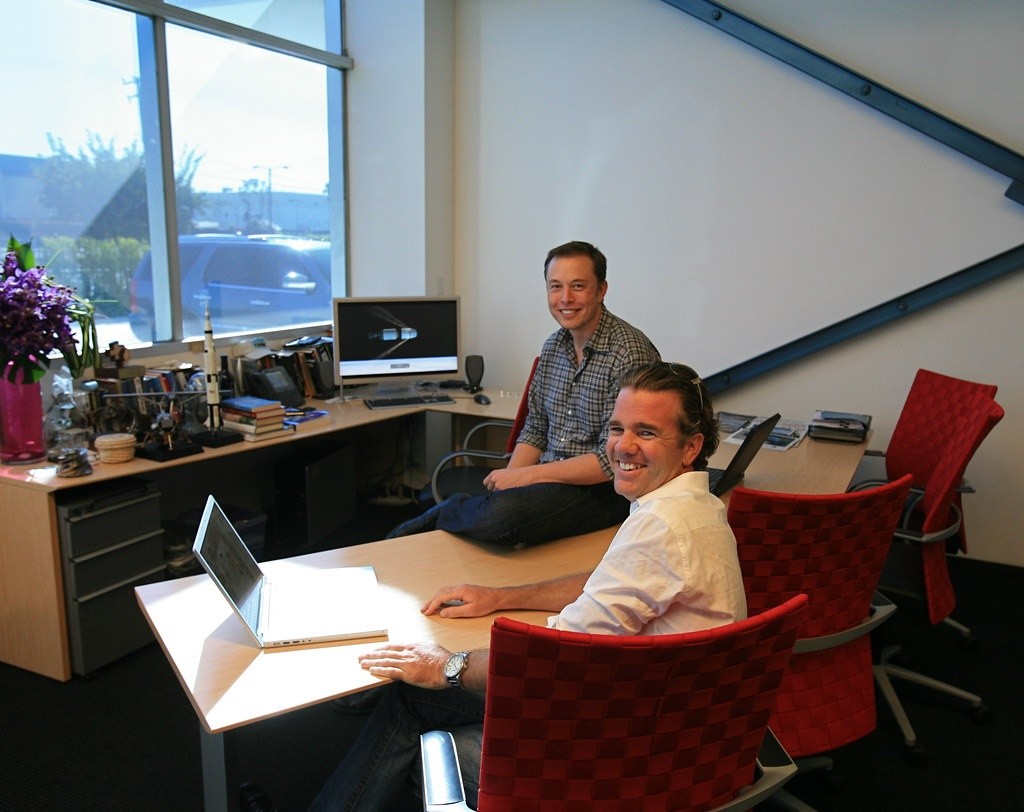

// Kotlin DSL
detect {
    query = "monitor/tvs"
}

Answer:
[332,297,461,386]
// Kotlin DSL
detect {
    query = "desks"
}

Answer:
[0,384,875,812]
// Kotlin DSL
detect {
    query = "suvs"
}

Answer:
[126,231,334,345]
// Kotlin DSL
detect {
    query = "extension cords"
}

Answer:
[375,494,413,506]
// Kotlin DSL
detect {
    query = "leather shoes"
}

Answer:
[384,497,450,542]
[329,689,381,714]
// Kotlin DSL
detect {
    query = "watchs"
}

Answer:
[446,650,472,690]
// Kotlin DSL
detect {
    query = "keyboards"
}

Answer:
[363,394,456,410]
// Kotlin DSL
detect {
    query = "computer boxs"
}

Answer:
[271,440,356,549]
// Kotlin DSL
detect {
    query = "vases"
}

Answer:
[0,352,49,464]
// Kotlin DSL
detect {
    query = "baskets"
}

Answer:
[95,433,135,463]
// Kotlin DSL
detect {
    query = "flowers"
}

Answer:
[0,233,89,385]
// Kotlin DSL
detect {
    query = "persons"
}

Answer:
[238,362,749,811]
[386,240,663,554]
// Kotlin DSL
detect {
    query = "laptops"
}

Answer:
[707,413,782,497]
[191,494,389,646]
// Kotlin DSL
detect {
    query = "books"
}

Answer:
[94,329,334,443]
[722,408,872,453]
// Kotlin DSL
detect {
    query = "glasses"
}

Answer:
[654,361,703,411]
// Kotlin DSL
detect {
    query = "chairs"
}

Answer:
[421,355,1006,812]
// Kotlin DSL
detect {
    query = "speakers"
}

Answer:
[462,355,484,393]
[310,362,335,400]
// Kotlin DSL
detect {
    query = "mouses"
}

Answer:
[474,394,490,405]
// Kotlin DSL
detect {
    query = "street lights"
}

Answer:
[252,163,290,231]
